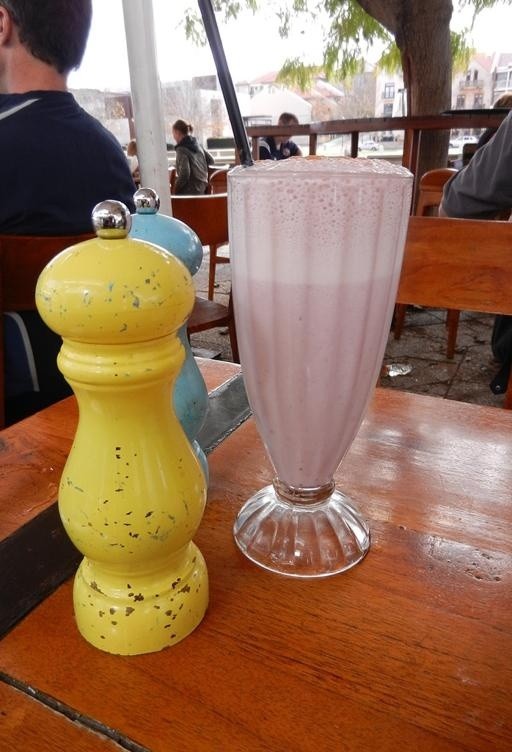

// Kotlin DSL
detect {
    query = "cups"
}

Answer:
[226,158,415,579]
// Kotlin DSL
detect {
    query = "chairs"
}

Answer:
[0,167,512,431]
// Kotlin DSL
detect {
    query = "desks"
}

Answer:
[0,359,512,752]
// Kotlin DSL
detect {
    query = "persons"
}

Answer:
[474,94,512,148]
[259,113,302,162]
[490,313,511,394]
[171,120,215,195]
[1,0,138,438]
[437,110,511,218]
[125,139,138,175]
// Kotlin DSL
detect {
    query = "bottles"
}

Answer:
[32,200,211,657]
[129,187,210,486]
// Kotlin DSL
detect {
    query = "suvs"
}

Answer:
[448,135,479,148]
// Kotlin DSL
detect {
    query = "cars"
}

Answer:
[357,140,384,153]
[382,130,394,141]
